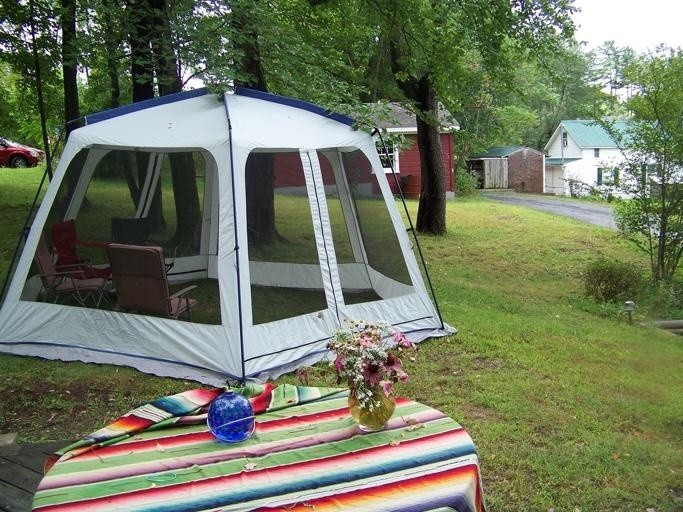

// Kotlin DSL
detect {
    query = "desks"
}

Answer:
[34,381,478,512]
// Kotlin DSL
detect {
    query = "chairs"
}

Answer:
[22,219,199,320]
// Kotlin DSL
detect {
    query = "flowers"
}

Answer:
[296,317,421,412]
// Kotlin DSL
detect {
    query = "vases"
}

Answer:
[347,386,396,432]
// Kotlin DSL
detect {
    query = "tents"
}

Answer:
[1,85,458,393]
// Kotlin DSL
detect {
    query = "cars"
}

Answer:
[0,136,45,168]
